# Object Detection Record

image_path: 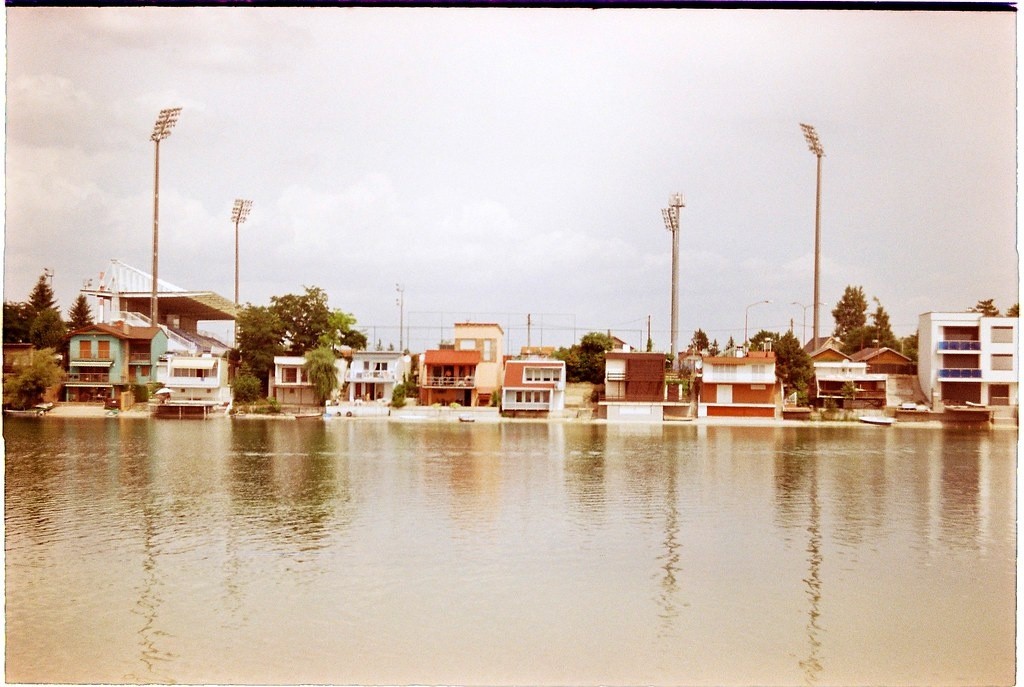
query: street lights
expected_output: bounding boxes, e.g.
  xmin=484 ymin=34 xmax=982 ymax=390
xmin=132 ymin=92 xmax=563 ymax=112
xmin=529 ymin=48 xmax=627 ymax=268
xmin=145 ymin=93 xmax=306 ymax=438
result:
xmin=662 ymin=191 xmax=687 ymax=373
xmin=792 ymin=302 xmax=831 ymax=347
xmin=146 ymin=105 xmax=183 ymax=326
xmin=798 ymin=119 xmax=828 ymax=355
xmin=744 ymin=300 xmax=773 ymax=356
xmin=394 ymin=281 xmax=406 ymax=353
xmin=231 ymin=197 xmax=255 ymax=309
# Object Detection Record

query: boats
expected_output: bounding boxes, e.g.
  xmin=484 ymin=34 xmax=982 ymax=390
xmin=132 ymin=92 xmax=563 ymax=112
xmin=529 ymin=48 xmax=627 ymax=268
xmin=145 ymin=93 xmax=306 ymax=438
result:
xmin=859 ymin=414 xmax=898 ymax=426
